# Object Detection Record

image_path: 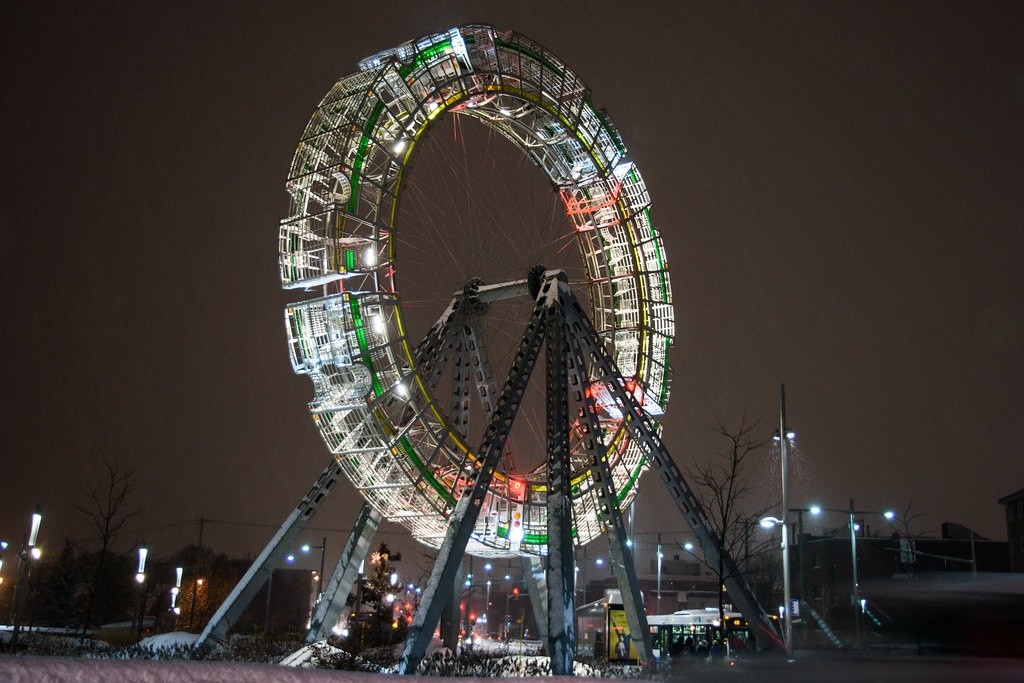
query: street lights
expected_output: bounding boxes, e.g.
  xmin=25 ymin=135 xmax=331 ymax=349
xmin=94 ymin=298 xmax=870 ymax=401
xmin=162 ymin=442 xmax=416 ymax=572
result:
xmin=656 ymin=533 xmax=665 ymax=616
xmin=755 ymin=380 xmax=796 ymax=663
xmin=9 ymin=532 xmax=43 ymax=655
xmin=128 ymin=557 xmax=152 ymax=645
xmin=188 ymin=570 xmax=204 ymax=634
xmin=808 ymin=499 xmax=898 ymax=643
xmin=302 ymin=537 xmax=328 ymax=602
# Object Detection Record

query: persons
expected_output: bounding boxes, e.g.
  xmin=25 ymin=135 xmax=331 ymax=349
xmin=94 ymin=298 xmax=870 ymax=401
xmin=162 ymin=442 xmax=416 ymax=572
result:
xmin=673 ymin=635 xmax=745 ymax=661
xmin=610 ymin=616 xmax=633 ymax=660
